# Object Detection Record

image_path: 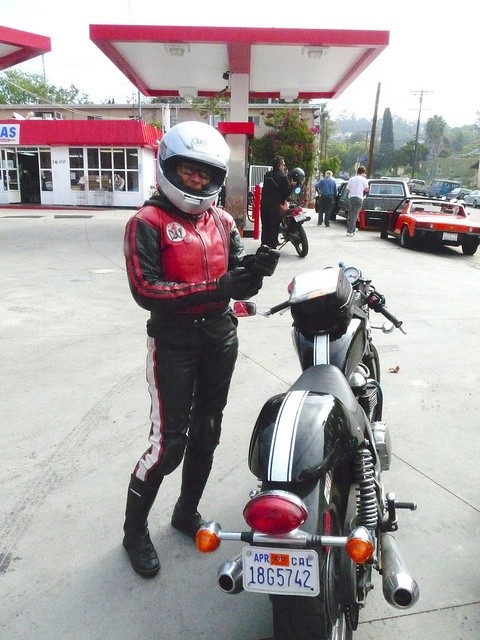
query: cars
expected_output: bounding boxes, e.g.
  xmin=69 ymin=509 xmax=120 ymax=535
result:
xmin=380 ymin=196 xmax=480 ymax=255
xmin=463 ymin=190 xmax=480 ymax=208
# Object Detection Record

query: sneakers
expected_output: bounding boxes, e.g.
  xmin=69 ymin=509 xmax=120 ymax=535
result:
xmin=353 ymin=228 xmax=358 ymax=235
xmin=317 ymin=223 xmax=322 ymax=225
xmin=326 ymin=226 xmax=330 ymax=227
xmin=346 ymin=233 xmax=353 ymax=237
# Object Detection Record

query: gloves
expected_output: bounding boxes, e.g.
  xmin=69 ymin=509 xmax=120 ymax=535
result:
xmin=216 ymin=267 xmax=264 ymax=301
xmin=243 ymin=245 xmax=281 ymax=276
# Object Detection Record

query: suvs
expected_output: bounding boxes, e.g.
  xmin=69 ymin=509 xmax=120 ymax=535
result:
xmin=330 ymin=179 xmax=410 ymax=228
xmin=408 ymin=179 xmax=426 ymax=196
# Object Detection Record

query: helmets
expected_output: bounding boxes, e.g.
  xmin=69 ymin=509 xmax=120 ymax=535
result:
xmin=289 ymin=167 xmax=305 ymax=187
xmin=156 ymin=121 xmax=231 ymax=214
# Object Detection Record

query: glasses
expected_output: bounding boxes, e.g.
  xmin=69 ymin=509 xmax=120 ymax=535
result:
xmin=179 ymin=161 xmax=214 ymax=180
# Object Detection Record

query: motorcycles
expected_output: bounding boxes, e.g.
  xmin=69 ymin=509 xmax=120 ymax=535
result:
xmin=278 ymin=186 xmax=311 ymax=258
xmin=196 ymin=262 xmax=420 ymax=636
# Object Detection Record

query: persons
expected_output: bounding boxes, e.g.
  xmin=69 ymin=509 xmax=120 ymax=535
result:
xmin=114 ymin=174 xmax=124 ymax=191
xmin=315 ymin=171 xmax=338 ymax=227
xmin=123 ymin=121 xmax=280 ymax=577
xmin=346 ymin=167 xmax=369 ymax=237
xmin=261 ymin=154 xmax=297 ymax=252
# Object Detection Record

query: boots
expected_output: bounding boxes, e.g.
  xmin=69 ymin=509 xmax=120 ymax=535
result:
xmin=123 ymin=474 xmax=164 ymax=576
xmin=171 ymin=447 xmax=214 ymax=538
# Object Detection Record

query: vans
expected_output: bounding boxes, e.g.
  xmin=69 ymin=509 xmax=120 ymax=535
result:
xmin=428 ymin=180 xmax=459 ymax=199
